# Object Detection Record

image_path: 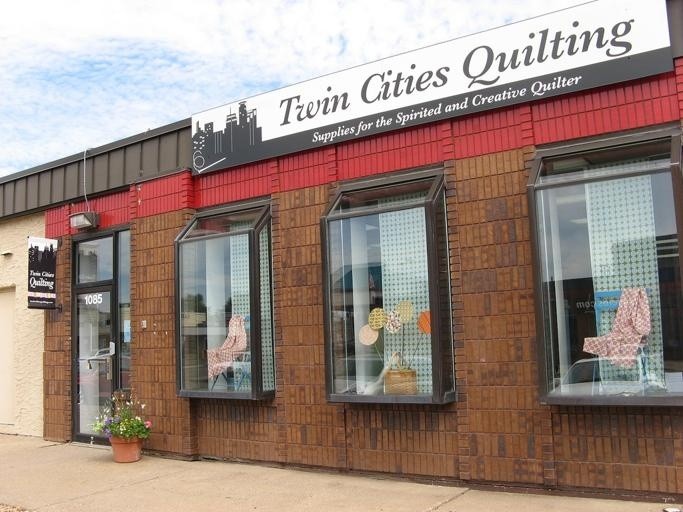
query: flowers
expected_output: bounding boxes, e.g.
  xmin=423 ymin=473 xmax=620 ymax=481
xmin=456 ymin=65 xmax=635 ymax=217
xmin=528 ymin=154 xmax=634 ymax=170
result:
xmin=92 ymin=395 xmax=152 ymax=441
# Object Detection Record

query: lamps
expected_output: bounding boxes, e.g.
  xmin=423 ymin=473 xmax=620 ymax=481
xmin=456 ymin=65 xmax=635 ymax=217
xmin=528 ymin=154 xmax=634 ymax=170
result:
xmin=68 ymin=211 xmax=96 ymax=232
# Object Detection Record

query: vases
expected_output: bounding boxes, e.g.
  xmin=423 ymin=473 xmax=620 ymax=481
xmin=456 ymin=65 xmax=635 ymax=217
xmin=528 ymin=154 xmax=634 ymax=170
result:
xmin=109 ymin=433 xmax=142 ymax=463
